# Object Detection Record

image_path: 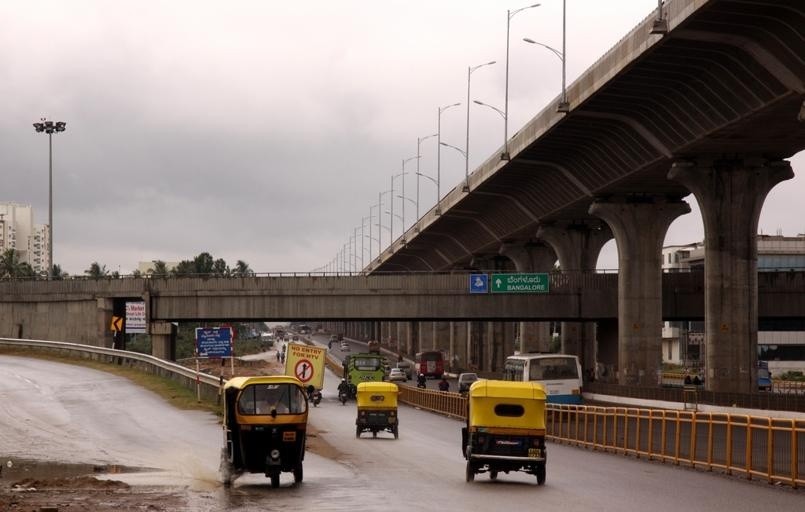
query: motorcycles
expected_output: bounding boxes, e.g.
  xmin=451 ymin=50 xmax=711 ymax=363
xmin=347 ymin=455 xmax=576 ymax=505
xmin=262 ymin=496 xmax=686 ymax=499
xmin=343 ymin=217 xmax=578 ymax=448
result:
xmin=337 ymin=387 xmax=347 ymax=405
xmin=312 ymin=390 xmax=322 ymax=407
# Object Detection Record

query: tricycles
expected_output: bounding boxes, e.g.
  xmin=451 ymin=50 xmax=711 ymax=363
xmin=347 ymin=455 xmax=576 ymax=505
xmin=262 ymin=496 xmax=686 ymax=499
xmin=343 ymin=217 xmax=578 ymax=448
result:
xmin=461 ymin=378 xmax=547 ymax=486
xmin=355 ymin=381 xmax=402 ymax=440
xmin=218 ymin=376 xmax=309 ymax=489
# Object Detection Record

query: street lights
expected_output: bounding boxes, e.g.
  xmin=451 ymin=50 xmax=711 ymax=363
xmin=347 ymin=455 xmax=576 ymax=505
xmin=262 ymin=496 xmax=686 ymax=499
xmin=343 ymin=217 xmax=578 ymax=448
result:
xmin=32 ymin=120 xmax=66 ymax=280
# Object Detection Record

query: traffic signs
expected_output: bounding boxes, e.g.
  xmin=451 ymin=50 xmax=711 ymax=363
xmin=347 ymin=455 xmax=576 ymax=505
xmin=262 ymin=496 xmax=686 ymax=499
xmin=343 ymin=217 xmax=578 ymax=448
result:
xmin=285 ymin=343 xmax=326 ymax=390
xmin=490 ymin=272 xmax=551 ymax=294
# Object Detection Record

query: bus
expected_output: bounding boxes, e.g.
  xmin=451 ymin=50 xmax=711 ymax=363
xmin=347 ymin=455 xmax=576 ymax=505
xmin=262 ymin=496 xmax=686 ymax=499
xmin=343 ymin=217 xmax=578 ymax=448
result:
xmin=501 ymin=353 xmax=583 ymax=418
xmin=342 ymin=352 xmax=385 ymax=400
xmin=415 ymin=349 xmax=444 ymax=380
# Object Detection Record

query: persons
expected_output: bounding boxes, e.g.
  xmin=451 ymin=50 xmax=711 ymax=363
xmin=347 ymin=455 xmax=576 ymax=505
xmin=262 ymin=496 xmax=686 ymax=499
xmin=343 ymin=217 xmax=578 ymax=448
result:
xmin=439 ymin=377 xmax=449 ymax=391
xmin=276 ymin=336 xmax=286 ymax=363
xmin=685 ymin=375 xmax=692 ymax=385
xmin=693 ymin=376 xmax=701 ymax=384
xmin=585 ymin=368 xmax=596 ymax=382
xmin=417 ymin=374 xmax=427 ymax=389
xmin=699 ymin=369 xmax=704 ymax=377
xmin=337 ymin=377 xmax=347 ymax=397
xmin=683 ymin=368 xmax=689 ymax=376
xmin=258 ymin=389 xmax=288 ymax=414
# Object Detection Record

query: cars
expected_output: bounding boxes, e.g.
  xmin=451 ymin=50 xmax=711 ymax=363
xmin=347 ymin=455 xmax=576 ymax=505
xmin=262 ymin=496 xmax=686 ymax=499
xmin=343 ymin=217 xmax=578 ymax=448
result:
xmin=383 ymin=356 xmax=413 ymax=383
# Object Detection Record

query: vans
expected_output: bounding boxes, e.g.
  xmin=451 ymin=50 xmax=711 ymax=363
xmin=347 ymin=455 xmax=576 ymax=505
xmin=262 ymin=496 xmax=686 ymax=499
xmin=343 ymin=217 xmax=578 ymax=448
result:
xmin=340 ymin=339 xmax=350 ymax=352
xmin=368 ymin=340 xmax=380 ymax=356
xmin=329 ymin=335 xmax=338 ymax=343
xmin=458 ymin=372 xmax=479 ymax=394
